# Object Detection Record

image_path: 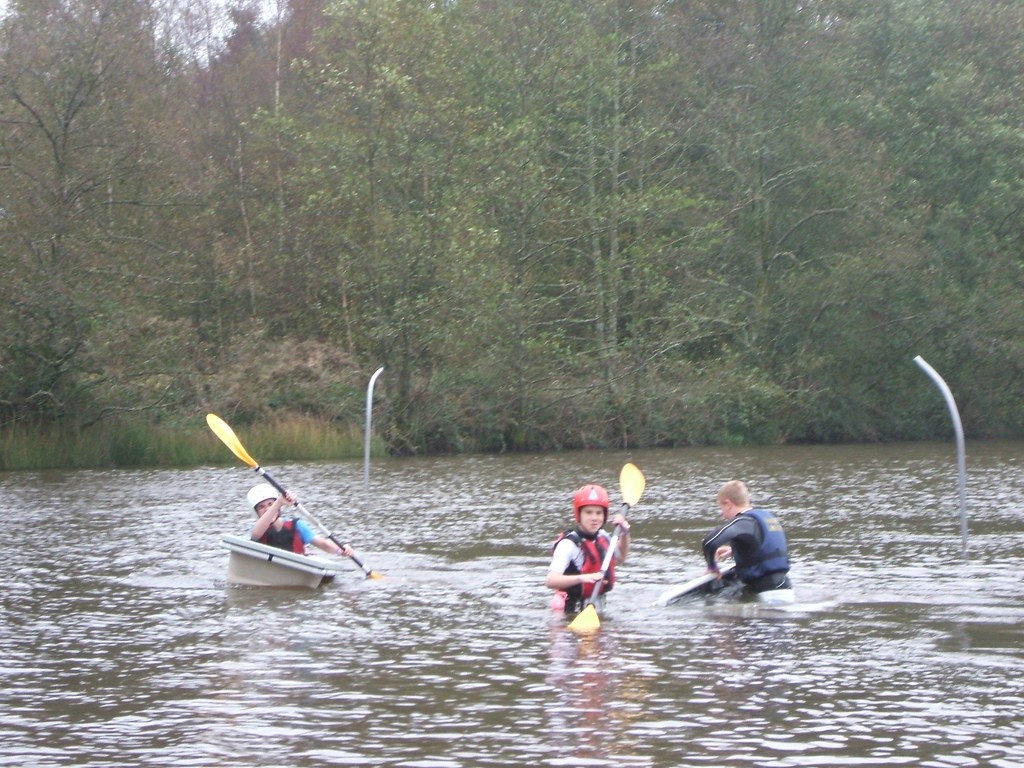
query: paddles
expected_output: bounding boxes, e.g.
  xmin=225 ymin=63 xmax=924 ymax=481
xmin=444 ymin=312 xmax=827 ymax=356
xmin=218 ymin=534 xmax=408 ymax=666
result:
xmin=567 ymin=463 xmax=646 ymax=635
xmin=205 ymin=411 xmax=381 ymax=579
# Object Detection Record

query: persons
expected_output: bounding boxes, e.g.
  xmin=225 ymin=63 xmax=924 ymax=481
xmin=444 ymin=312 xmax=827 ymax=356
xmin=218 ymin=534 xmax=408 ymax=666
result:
xmin=701 ymin=479 xmax=794 ymax=593
xmin=544 ymin=483 xmax=632 ymax=611
xmin=246 ymin=483 xmax=354 ymax=558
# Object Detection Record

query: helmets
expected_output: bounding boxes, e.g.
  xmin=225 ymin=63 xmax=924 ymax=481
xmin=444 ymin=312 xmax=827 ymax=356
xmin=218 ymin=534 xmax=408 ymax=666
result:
xmin=574 ymin=484 xmax=610 ymax=523
xmin=247 ymin=484 xmax=279 ymax=520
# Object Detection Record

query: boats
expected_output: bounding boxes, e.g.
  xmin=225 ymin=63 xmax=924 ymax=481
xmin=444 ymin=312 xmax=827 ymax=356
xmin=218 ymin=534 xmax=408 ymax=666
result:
xmin=647 ymin=559 xmax=837 ymax=618
xmin=218 ymin=533 xmax=344 ymax=592
